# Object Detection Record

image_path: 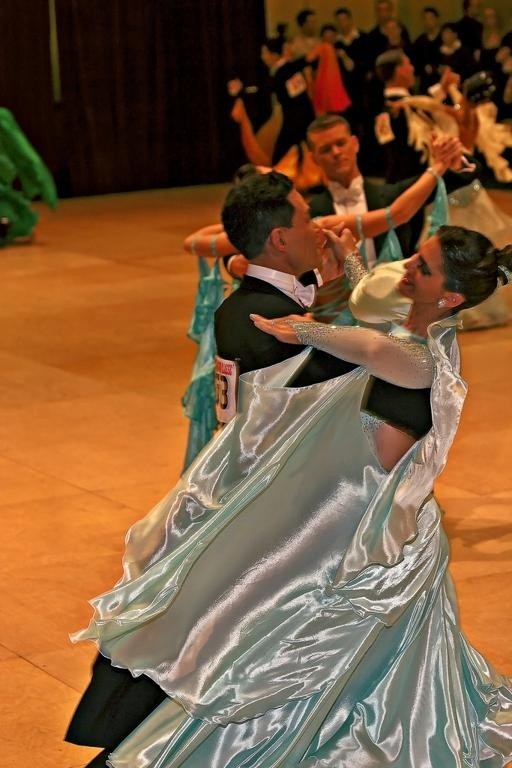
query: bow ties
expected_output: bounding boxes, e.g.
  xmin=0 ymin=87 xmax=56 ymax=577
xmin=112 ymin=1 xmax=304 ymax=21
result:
xmin=295 ymin=283 xmax=315 ymax=309
xmin=332 ymin=187 xmax=362 ymax=204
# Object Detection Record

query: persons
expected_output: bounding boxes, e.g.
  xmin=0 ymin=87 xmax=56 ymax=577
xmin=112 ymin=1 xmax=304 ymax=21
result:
xmin=181 ymin=1 xmax=511 ymax=473
xmin=63 ymin=172 xmax=346 ymax=766
xmin=69 ymin=223 xmax=511 ymax=766
